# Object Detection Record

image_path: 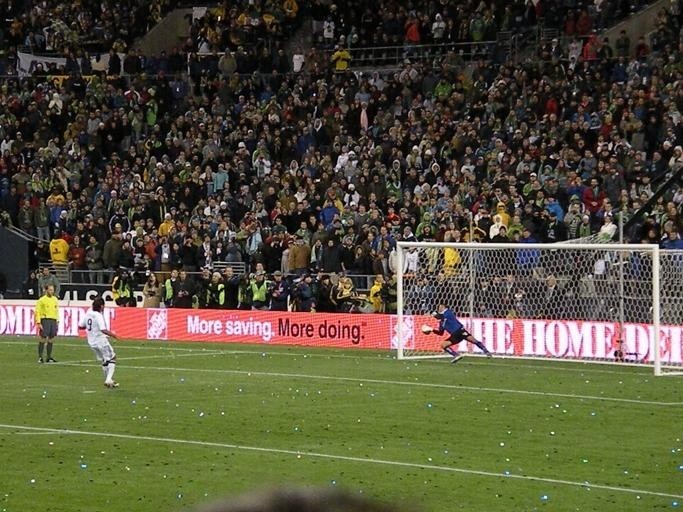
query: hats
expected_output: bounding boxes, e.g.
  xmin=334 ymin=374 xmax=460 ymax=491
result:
xmin=135 ymin=238 xmax=145 ymax=243
xmin=165 ymin=212 xmax=171 ymax=219
xmin=256 ymin=271 xmax=265 ymax=276
xmin=412 ymin=145 xmax=419 ymax=151
xmin=425 ymin=149 xmax=433 ymax=156
xmin=274 ymin=270 xmax=282 ymax=277
xmin=113 ymin=223 xmax=123 ymax=229
xmin=237 ymin=142 xmax=246 ymax=148
xmin=350 ymin=201 xmax=356 ymax=206
xmin=529 ymin=172 xmax=537 ymax=177
xmin=220 ymin=201 xmax=227 ymax=207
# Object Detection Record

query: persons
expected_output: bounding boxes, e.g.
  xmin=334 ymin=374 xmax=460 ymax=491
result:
xmin=0 ymin=2 xmax=682 ymax=324
xmin=77 ymin=298 xmax=124 ymax=388
xmin=34 ymin=283 xmax=60 ymax=364
xmin=428 ymin=303 xmax=492 ymax=364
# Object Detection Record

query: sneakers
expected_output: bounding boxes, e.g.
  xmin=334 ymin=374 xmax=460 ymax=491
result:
xmin=46 ymin=358 xmax=57 ymax=362
xmin=104 ymin=382 xmax=113 ymax=389
xmin=113 ymin=381 xmax=119 ymax=387
xmin=486 ymin=352 xmax=491 ymax=358
xmin=38 ymin=357 xmax=43 ymax=363
xmin=450 ymin=354 xmax=463 ymax=364
